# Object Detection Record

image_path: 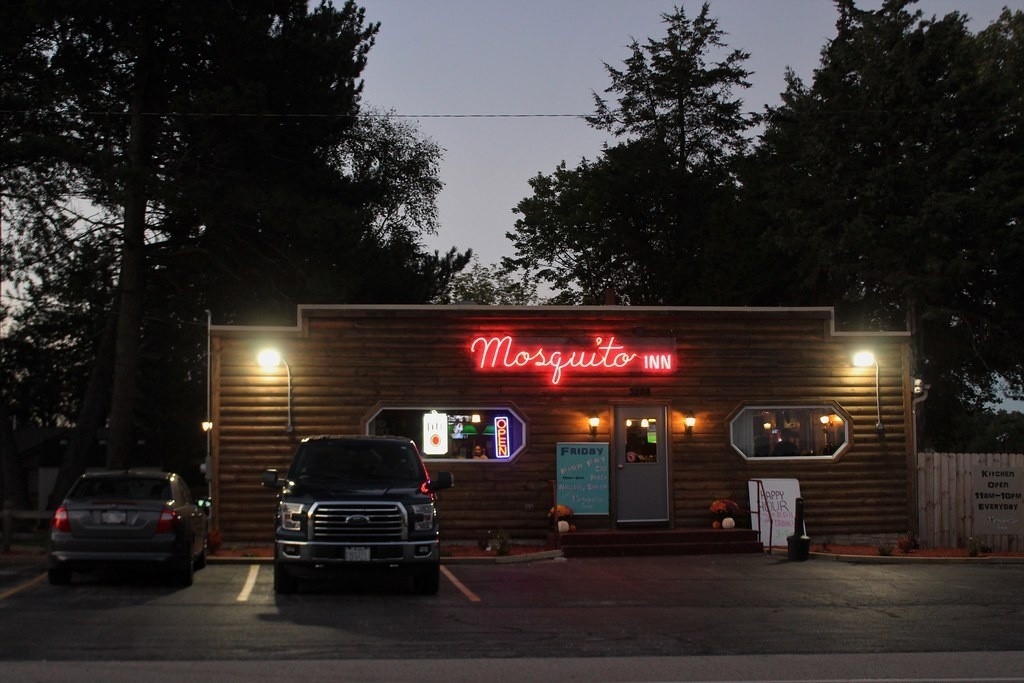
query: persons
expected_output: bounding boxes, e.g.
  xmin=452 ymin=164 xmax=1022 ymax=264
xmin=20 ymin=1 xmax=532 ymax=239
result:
xmin=769 ymin=428 xmax=799 ymax=456
xmin=472 ymin=445 xmax=488 ymax=460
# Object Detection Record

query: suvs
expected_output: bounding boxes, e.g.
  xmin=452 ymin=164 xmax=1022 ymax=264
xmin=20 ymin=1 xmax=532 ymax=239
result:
xmin=262 ymin=434 xmax=455 ymax=600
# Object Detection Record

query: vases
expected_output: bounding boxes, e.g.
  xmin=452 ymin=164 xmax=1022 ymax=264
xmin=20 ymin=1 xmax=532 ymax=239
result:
xmin=558 ymin=521 xmax=569 ymax=532
xmin=722 ymin=518 xmax=735 ymax=529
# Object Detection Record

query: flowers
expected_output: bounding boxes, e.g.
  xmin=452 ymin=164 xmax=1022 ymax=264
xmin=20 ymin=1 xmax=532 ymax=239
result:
xmin=549 ymin=506 xmax=573 ymax=519
xmin=710 ymin=499 xmax=738 ymax=515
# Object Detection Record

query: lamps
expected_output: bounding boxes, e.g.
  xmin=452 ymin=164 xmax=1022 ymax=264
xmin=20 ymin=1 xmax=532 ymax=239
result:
xmin=257 ymin=348 xmax=293 ymax=432
xmin=683 ymin=406 xmax=696 ymax=436
xmin=852 ymin=348 xmax=883 ymax=429
xmin=588 ymin=410 xmax=600 ymax=438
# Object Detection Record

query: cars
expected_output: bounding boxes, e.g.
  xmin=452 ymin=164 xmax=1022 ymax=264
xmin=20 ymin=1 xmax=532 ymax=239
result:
xmin=47 ymin=466 xmax=209 ymax=591
